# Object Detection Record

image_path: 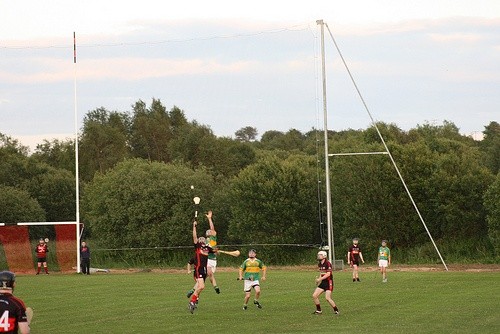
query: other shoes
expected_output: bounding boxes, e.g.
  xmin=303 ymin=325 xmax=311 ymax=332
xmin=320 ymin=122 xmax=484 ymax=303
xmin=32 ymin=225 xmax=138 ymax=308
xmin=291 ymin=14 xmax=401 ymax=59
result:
xmin=214 ymin=285 xmax=221 ymax=294
xmin=254 ymin=299 xmax=263 ymax=308
xmin=36 ymin=273 xmax=39 ymax=275
xmin=46 ymin=272 xmax=49 ymax=275
xmin=243 ymin=305 xmax=247 ymax=310
xmin=382 ymin=277 xmax=387 ymax=282
xmin=353 ymin=279 xmax=356 ymax=282
xmin=357 ymin=278 xmax=359 ymax=281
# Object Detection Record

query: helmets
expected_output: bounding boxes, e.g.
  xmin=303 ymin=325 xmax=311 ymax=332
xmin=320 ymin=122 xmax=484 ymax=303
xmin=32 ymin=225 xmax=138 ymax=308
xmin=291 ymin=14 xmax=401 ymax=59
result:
xmin=248 ymin=248 xmax=257 ymax=258
xmin=318 ymin=251 xmax=327 ymax=260
xmin=205 ymin=229 xmax=212 ymax=237
xmin=0 ymin=271 xmax=15 ymax=291
xmin=198 ymin=236 xmax=206 ymax=244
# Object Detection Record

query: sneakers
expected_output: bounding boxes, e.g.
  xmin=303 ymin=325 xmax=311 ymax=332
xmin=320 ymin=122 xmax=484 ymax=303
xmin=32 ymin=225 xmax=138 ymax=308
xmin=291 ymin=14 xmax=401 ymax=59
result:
xmin=186 ymin=288 xmax=195 ymax=298
xmin=188 ymin=301 xmax=198 ymax=314
xmin=313 ymin=310 xmax=323 ymax=314
xmin=334 ymin=310 xmax=338 ymax=314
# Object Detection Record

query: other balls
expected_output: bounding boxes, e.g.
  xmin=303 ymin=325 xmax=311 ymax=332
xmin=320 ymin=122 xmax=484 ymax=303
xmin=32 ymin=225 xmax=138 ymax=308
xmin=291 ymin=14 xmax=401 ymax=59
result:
xmin=191 ymin=185 xmax=194 ymax=189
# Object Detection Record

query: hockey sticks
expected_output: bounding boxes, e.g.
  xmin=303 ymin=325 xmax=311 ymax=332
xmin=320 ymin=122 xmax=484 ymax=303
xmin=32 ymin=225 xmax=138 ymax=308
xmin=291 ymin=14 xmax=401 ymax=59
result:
xmin=213 ymin=249 xmax=240 ymax=256
xmin=25 ymin=306 xmax=34 ymax=326
xmin=193 ymin=196 xmax=201 ymax=227
xmin=347 ymin=261 xmax=353 ymax=270
xmin=41 ymin=238 xmax=49 ymax=250
xmin=237 ymin=275 xmax=255 ymax=281
xmin=315 ymin=276 xmax=321 ymax=286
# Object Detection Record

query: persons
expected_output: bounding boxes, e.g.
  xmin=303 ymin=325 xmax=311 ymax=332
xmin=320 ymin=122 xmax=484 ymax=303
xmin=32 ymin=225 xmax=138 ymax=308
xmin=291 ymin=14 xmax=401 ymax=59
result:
xmin=187 ymin=211 xmax=220 ymax=298
xmin=80 ymin=241 xmax=90 ymax=275
xmin=188 ymin=221 xmax=220 ymax=314
xmin=239 ymin=249 xmax=266 ymax=311
xmin=0 ymin=271 xmax=31 ymax=334
xmin=348 ymin=238 xmax=364 ymax=283
xmin=377 ymin=240 xmax=390 ymax=282
xmin=35 ymin=237 xmax=50 ymax=275
xmin=311 ymin=251 xmax=340 ymax=316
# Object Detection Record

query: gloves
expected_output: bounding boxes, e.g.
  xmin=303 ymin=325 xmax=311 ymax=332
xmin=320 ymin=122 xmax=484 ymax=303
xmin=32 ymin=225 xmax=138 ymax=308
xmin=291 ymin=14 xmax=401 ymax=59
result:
xmin=25 ymin=307 xmax=34 ymax=325
